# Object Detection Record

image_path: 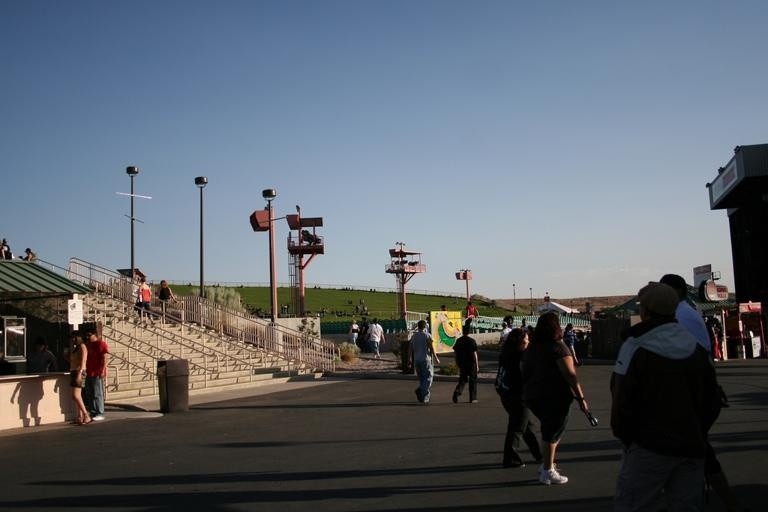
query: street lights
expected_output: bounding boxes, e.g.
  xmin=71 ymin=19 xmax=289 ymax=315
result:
xmin=261 ymin=188 xmax=277 ymax=324
xmin=193 ymin=175 xmax=209 ymax=298
xmin=529 ymin=287 xmax=534 ymax=316
xmin=126 ymin=166 xmax=139 ymax=278
xmin=512 ymin=283 xmax=517 ymax=313
xmin=392 ymin=260 xmax=419 ymax=322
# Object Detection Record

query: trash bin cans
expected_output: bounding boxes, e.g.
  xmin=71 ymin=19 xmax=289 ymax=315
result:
xmin=400 ymin=340 xmax=415 ymax=374
xmin=156 ymin=359 xmax=189 ymax=412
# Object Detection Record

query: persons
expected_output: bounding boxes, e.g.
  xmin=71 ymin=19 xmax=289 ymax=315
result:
xmin=25 ymin=245 xmax=38 ymax=264
xmin=344 ymin=294 xmax=612 ymax=369
xmin=60 ymin=332 xmax=92 ymax=427
xmin=452 ymin=324 xmax=484 ymax=404
xmin=157 ymin=279 xmax=179 ymax=321
xmin=25 ymin=335 xmax=59 ymax=372
xmin=2 ymin=237 xmax=13 ymax=262
xmin=405 ymin=319 xmax=442 ymax=407
xmin=133 ymin=277 xmax=156 ymax=329
xmin=608 ymin=281 xmax=724 ymax=511
xmin=659 ymin=271 xmax=740 ymax=511
xmin=84 ymin=327 xmax=110 ymax=422
xmin=492 ymin=327 xmax=543 ymax=469
xmin=521 ymin=309 xmax=599 ymax=487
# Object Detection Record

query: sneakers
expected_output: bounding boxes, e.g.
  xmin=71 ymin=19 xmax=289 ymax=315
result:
xmin=537 ymin=462 xmax=569 ymax=485
xmin=93 ymin=416 xmax=106 ymax=421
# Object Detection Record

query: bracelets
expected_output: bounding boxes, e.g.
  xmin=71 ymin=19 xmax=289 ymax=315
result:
xmin=580 ymin=397 xmax=585 ymax=402
